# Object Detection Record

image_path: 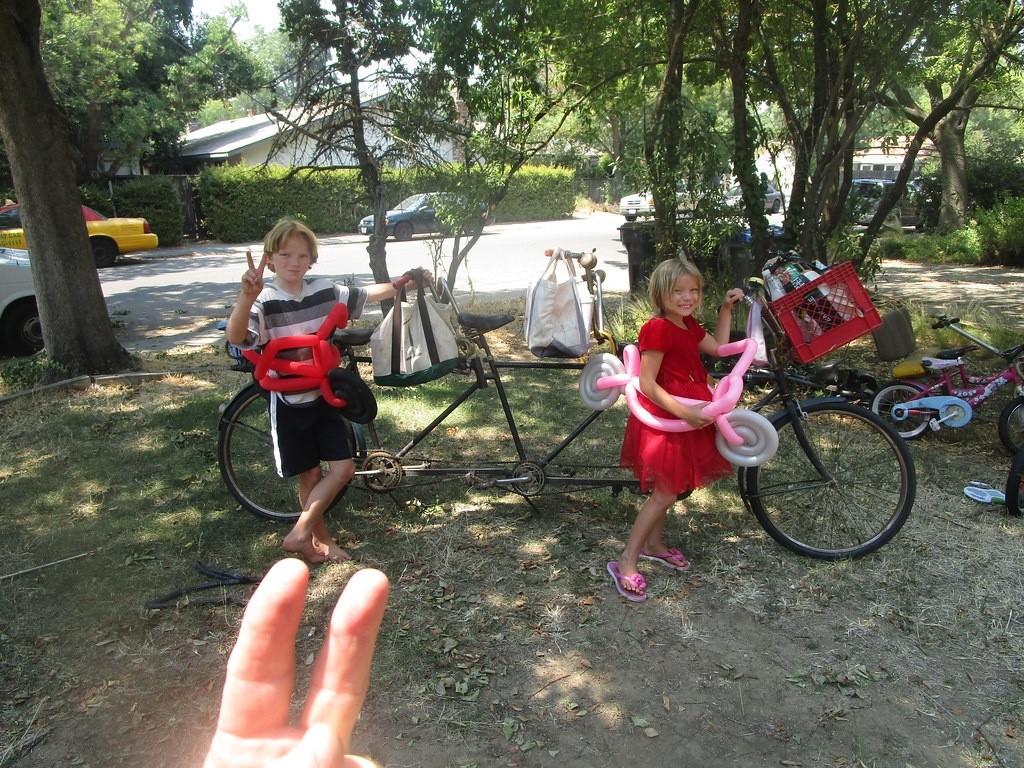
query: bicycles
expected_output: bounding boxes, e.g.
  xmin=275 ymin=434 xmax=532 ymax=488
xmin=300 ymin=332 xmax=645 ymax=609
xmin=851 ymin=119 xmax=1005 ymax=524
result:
xmin=213 ymin=268 xmax=919 ymax=560
xmin=869 ymin=343 xmax=1024 ymax=519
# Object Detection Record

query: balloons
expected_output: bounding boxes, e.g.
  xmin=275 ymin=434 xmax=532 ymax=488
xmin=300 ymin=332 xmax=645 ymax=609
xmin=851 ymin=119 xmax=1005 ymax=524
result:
xmin=579 ymin=338 xmax=779 ymax=468
xmin=241 ymin=302 xmax=377 ymax=425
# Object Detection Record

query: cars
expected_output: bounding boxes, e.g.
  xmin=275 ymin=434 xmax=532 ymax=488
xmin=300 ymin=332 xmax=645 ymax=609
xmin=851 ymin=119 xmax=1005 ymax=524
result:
xmin=619 ymin=176 xmax=722 ymax=221
xmin=0 ymin=197 xmax=160 ymax=269
xmin=726 ymin=183 xmax=784 ymax=217
xmin=359 ymin=189 xmax=496 ymax=243
xmin=0 ymin=247 xmax=47 ymax=359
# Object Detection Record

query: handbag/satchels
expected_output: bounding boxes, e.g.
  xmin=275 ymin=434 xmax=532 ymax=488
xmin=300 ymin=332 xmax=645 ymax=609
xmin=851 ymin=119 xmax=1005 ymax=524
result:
xmin=522 ymin=247 xmax=594 ymax=357
xmin=371 ymin=269 xmax=460 ymax=386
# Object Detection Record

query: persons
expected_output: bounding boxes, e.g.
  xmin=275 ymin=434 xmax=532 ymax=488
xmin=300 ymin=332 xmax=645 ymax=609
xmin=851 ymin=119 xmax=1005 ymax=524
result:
xmin=226 ymin=220 xmax=436 ymax=563
xmin=201 ymin=557 xmax=390 ymax=768
xmin=618 ymin=260 xmax=746 ymax=595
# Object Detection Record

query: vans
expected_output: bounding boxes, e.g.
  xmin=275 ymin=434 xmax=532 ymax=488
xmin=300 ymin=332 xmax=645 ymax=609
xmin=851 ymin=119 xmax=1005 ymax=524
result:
xmin=843 ymin=177 xmax=930 ymax=234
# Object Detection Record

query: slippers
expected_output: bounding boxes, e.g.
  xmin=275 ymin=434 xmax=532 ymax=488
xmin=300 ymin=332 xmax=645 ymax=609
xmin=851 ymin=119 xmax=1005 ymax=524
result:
xmin=638 ymin=547 xmax=690 ymax=571
xmin=606 ymin=561 xmax=647 ymax=602
